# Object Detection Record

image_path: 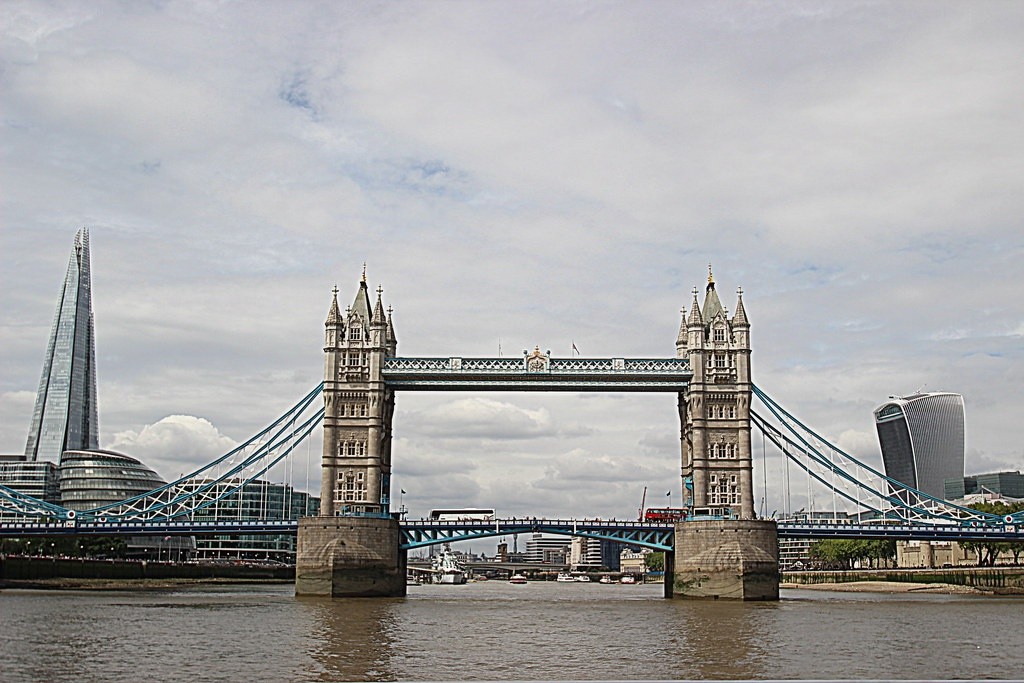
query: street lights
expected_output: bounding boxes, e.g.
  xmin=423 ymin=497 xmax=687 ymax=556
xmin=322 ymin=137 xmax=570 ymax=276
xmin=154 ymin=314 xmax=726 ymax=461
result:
xmin=27 ymin=541 xmax=32 ymax=558
xmin=51 ymin=542 xmax=55 ymax=559
xmin=143 ymin=549 xmax=290 ymax=564
xmin=111 ymin=547 xmax=115 ymax=562
xmin=80 ymin=545 xmax=84 ymax=560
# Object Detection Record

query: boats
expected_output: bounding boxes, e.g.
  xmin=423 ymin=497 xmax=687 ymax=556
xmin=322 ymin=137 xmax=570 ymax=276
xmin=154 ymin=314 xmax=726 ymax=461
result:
xmin=432 ymin=545 xmax=468 ymax=584
xmin=556 ymin=572 xmax=636 ymax=584
xmin=509 ymin=573 xmax=527 ymax=584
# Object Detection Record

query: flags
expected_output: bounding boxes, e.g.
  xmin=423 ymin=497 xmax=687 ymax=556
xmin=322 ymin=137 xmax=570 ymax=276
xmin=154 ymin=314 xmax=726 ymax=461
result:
xmin=667 ymin=492 xmax=670 ymax=496
xmin=573 ymin=344 xmax=579 ymax=354
xmin=401 ymin=489 xmax=406 ymax=494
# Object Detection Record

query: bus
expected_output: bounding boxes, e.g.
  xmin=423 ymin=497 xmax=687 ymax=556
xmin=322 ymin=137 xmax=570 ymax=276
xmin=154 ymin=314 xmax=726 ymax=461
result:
xmin=645 ymin=507 xmax=690 ymax=523
xmin=428 ymin=508 xmax=496 ymax=525
